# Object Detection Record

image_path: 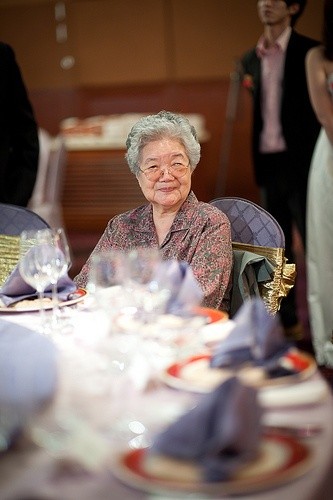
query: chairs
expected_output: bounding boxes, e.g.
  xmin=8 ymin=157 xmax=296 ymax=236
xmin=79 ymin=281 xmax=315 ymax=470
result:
xmin=207 ymin=196 xmax=286 ymax=321
xmin=0 ymin=204 xmax=52 ymax=289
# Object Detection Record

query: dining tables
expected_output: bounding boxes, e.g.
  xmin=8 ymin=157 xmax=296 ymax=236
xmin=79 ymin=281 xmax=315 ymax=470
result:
xmin=0 ymin=292 xmax=333 ymax=500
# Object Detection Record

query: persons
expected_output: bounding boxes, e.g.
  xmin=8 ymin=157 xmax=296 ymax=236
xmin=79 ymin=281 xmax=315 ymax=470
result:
xmin=72 ymin=110 xmax=232 ymax=311
xmin=0 ymin=42 xmax=40 ymax=208
xmin=240 ymin=0 xmax=321 ymax=327
xmin=306 ymin=0 xmax=333 ymax=365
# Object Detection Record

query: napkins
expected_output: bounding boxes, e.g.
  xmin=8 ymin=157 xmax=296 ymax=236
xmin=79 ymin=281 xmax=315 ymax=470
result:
xmin=149 ymin=258 xmax=206 ymax=316
xmin=207 ymin=296 xmax=296 ymax=367
xmin=148 ymin=373 xmax=272 ymax=483
xmin=0 ymin=241 xmax=77 ymax=306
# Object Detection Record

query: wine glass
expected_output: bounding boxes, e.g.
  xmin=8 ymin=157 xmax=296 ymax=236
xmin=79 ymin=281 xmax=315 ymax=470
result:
xmin=83 ymin=246 xmax=186 ymax=424
xmin=18 ymin=226 xmax=74 ymax=326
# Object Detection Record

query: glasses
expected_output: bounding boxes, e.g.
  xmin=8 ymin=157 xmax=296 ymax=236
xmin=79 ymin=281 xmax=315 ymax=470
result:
xmin=135 ymin=156 xmax=189 ymax=182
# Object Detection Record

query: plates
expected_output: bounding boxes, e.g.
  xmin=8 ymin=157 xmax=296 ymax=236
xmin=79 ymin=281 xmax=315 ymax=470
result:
xmin=196 ymin=306 xmax=229 ymax=326
xmin=161 ymin=346 xmax=318 ymax=396
xmin=106 ymin=431 xmax=317 ymax=496
xmin=1 ymin=285 xmax=85 ymax=313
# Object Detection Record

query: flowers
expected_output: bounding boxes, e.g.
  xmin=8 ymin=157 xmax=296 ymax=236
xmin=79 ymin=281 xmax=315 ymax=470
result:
xmin=243 ymin=74 xmax=253 ymax=89
